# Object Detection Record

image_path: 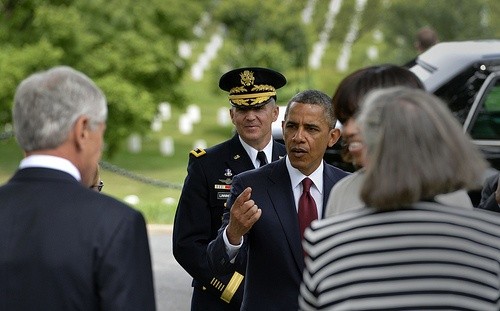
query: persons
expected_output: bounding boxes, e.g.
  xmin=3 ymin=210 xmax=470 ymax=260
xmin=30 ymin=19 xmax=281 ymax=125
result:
xmin=299 ymin=63 xmax=500 ymax=311
xmin=0 ymin=66 xmax=156 ymax=311
xmin=205 ymin=89 xmax=354 ymax=311
xmin=87 ymin=161 xmax=103 ymax=193
xmin=403 ymin=26 xmax=441 ymax=69
xmin=170 ymin=66 xmax=288 ymax=311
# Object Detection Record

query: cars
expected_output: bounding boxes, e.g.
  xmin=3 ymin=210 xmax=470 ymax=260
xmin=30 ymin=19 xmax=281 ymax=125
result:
xmin=272 ymin=38 xmax=500 ymax=209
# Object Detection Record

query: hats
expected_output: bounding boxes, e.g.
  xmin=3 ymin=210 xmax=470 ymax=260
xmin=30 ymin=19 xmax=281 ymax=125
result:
xmin=218 ymin=66 xmax=287 ymax=110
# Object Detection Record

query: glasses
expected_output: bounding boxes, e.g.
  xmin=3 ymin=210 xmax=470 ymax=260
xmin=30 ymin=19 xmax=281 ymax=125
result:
xmin=89 ymin=179 xmax=104 ymax=192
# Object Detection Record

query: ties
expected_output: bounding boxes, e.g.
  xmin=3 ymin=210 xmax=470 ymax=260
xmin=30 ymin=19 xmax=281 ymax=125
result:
xmin=256 ymin=151 xmax=268 ymax=169
xmin=297 ymin=178 xmax=319 ymax=258
